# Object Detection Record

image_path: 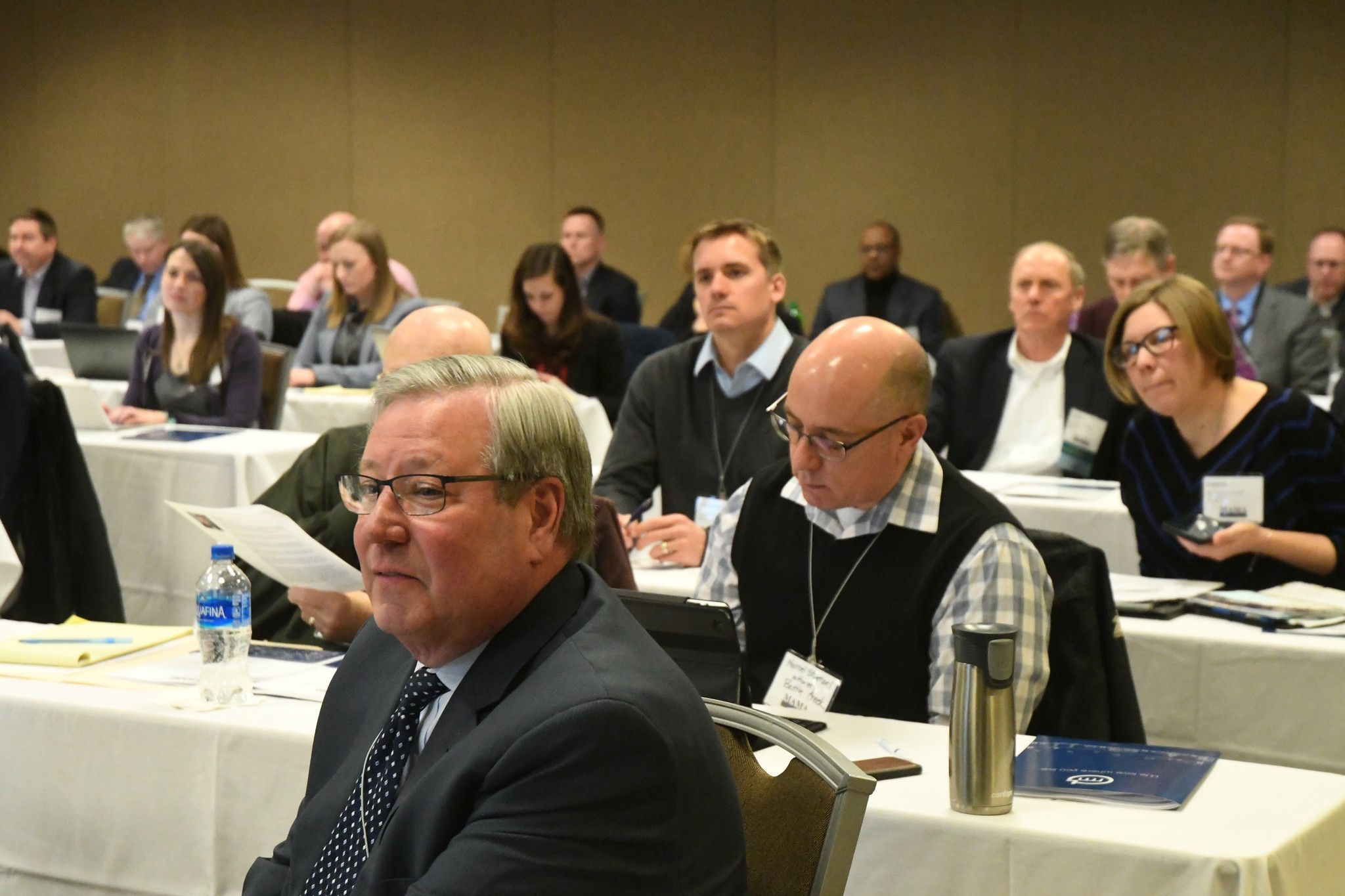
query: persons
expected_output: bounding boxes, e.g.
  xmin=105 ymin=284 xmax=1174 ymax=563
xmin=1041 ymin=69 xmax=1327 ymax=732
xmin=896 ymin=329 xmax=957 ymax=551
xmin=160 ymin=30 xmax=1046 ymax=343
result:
xmin=654 ymin=274 xmax=804 ymax=342
xmin=116 ymin=208 xmax=427 ymax=388
xmin=594 ymin=219 xmax=812 ymax=568
xmin=501 ymin=239 xmax=618 ymax=413
xmin=1078 ymin=214 xmax=1258 ymax=384
xmin=557 ymin=206 xmax=641 ymax=322
xmin=241 ymin=354 xmax=747 ymax=896
xmin=1102 ymin=273 xmax=1345 ymax=593
xmin=924 ymin=239 xmax=1127 ymax=484
xmin=690 ymin=315 xmax=1053 ymax=739
xmin=808 ymin=220 xmax=961 ymax=353
xmin=101 ymin=238 xmax=263 ymax=426
xmin=0 ymin=342 xmax=77 ymax=623
xmin=0 ymin=209 xmax=98 ymax=339
xmin=1206 ymin=216 xmax=1345 ymax=397
xmin=233 ymin=306 xmax=494 ymax=654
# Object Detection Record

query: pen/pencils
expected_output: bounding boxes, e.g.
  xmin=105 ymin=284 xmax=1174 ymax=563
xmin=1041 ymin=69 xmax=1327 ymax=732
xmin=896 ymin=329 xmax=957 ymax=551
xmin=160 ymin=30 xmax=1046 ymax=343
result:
xmin=1211 ymin=604 xmax=1273 ymax=626
xmin=622 ymin=498 xmax=654 ymax=529
xmin=18 ymin=636 xmax=131 ymax=643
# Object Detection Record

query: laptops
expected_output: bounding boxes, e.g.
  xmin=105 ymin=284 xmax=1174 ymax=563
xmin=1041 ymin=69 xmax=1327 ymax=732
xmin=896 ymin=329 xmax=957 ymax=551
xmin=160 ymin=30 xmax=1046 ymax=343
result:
xmin=614 ymin=587 xmax=826 ymax=752
xmin=61 ymin=323 xmax=140 ymax=380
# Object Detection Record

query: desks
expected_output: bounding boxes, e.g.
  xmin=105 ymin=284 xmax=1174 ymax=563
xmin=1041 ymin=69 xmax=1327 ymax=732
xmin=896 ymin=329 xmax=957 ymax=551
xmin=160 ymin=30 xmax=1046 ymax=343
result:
xmin=0 ymin=337 xmax=1345 ymax=896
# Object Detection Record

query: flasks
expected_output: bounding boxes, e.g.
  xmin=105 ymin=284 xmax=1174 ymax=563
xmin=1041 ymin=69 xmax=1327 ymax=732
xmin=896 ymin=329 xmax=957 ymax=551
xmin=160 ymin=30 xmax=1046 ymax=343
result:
xmin=948 ymin=621 xmax=1021 ymax=816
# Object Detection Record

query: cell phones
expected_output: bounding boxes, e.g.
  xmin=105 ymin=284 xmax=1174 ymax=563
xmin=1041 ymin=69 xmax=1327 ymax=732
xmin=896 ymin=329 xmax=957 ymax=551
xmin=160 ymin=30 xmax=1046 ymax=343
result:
xmin=1160 ymin=521 xmax=1227 ymax=542
xmin=854 ymin=757 xmax=922 ymax=781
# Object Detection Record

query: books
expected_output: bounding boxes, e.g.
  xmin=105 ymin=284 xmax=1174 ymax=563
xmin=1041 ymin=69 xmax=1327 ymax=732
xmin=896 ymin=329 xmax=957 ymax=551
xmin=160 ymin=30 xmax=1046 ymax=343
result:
xmin=1010 ymin=733 xmax=1222 ymax=810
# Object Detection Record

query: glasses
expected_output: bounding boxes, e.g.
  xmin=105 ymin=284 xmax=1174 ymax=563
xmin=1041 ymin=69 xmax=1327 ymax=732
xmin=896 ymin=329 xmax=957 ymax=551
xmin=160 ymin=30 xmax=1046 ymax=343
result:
xmin=1109 ymin=326 xmax=1178 ymax=369
xmin=766 ymin=391 xmax=910 ymax=463
xmin=335 ymin=473 xmax=528 ymax=517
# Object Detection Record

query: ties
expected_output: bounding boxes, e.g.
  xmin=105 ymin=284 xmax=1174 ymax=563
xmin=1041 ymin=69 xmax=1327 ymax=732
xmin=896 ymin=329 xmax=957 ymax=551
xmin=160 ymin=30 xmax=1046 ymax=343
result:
xmin=300 ymin=667 xmax=450 ymax=895
xmin=1224 ymin=308 xmax=1243 ymax=330
xmin=129 ymin=272 xmax=155 ymax=320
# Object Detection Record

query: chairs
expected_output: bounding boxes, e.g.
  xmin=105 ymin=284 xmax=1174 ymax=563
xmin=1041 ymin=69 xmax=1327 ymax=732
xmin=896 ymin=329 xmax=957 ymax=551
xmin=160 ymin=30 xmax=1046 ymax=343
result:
xmin=257 ymin=341 xmax=297 ymax=430
xmin=1024 ymin=528 xmax=1147 ymax=745
xmin=700 ymin=697 xmax=877 ymax=896
xmin=273 ymin=308 xmax=311 ymax=348
xmin=95 ymin=284 xmax=129 ymax=329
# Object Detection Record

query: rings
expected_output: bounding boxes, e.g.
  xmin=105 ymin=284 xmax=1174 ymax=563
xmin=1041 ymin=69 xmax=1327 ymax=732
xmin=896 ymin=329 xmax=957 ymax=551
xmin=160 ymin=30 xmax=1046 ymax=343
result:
xmin=307 ymin=617 xmax=315 ymax=626
xmin=661 ymin=542 xmax=669 ymax=554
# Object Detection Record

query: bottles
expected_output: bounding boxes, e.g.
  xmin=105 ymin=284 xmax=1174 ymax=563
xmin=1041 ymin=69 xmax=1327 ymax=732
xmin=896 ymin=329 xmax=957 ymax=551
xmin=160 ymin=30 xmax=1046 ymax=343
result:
xmin=787 ymin=301 xmax=803 ymax=327
xmin=194 ymin=544 xmax=255 ymax=707
